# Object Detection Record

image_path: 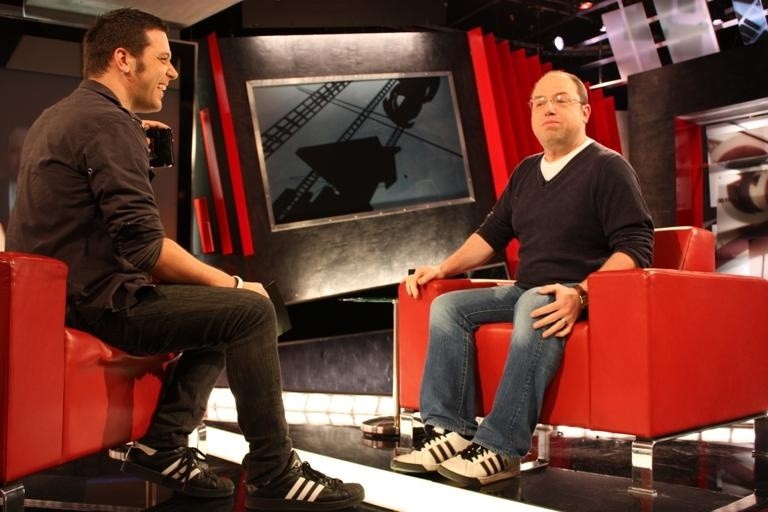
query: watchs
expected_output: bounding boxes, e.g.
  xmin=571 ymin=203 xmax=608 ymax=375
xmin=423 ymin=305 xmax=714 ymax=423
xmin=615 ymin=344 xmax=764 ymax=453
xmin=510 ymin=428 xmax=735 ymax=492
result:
xmin=570 ymin=284 xmax=588 ymax=310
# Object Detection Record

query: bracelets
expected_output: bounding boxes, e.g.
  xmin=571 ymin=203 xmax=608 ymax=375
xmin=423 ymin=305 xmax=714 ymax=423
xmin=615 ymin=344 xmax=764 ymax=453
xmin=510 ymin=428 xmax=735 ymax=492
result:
xmin=232 ymin=276 xmax=244 ymax=290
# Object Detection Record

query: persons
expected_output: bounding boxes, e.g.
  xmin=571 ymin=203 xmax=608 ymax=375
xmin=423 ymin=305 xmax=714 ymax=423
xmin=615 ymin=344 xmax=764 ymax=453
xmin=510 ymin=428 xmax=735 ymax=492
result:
xmin=390 ymin=70 xmax=655 ymax=487
xmin=4 ymin=8 xmax=364 ymax=511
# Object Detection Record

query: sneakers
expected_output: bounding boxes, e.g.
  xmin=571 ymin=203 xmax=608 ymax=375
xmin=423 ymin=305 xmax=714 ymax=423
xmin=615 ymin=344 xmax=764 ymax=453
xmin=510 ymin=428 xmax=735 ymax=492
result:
xmin=390 ymin=425 xmax=473 ymax=473
xmin=244 ymin=450 xmax=364 ymax=510
xmin=437 ymin=442 xmax=521 ymax=486
xmin=120 ymin=440 xmax=236 ymax=498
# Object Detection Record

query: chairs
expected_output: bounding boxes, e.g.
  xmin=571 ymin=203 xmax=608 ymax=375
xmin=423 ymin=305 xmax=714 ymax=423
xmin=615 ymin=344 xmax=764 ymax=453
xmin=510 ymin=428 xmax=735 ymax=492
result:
xmin=395 ymin=221 xmax=767 ymax=498
xmin=0 ymin=242 xmax=183 ymax=512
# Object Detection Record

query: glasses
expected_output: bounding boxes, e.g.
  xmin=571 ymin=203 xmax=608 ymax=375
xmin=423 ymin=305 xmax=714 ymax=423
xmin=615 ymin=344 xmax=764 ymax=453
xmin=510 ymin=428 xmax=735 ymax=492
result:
xmin=528 ymin=94 xmax=585 ymax=110
